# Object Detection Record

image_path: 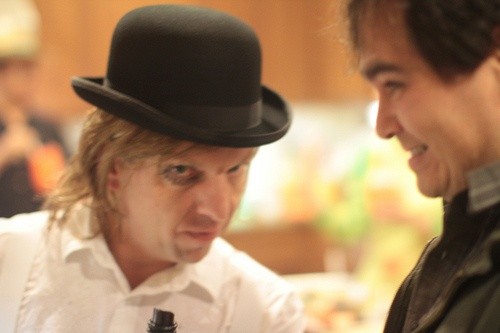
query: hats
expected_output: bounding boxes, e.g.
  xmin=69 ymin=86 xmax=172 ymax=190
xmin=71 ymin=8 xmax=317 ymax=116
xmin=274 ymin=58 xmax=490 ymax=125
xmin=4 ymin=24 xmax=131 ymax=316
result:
xmin=71 ymin=3 xmax=294 ymax=147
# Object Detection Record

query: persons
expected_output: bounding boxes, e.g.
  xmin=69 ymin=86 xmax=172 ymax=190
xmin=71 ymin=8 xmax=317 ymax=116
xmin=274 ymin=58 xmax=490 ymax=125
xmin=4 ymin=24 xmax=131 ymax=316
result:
xmin=0 ymin=1 xmax=75 ymax=218
xmin=2 ymin=6 xmax=308 ymax=333
xmin=345 ymin=0 xmax=500 ymax=333
